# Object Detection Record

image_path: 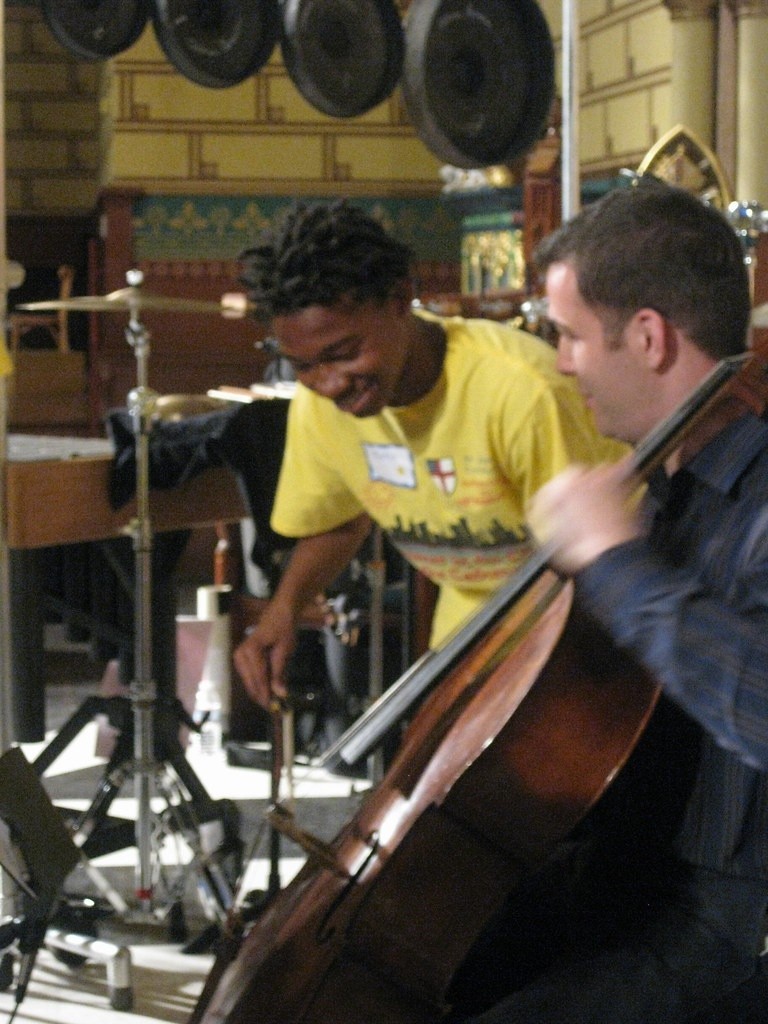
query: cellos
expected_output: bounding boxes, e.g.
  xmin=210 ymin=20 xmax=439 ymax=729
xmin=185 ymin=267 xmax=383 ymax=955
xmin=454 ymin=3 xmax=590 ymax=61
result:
xmin=191 ymin=313 xmax=767 ymax=1021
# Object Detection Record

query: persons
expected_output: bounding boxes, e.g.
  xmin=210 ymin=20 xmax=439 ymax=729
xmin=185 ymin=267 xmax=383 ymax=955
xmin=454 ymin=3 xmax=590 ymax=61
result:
xmin=432 ymin=178 xmax=768 ymax=1024
xmin=228 ymin=199 xmax=654 ymax=720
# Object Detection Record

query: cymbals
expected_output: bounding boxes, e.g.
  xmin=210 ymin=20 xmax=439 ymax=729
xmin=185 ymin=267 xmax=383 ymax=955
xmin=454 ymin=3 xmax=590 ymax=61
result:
xmin=16 ymin=287 xmax=236 ymax=312
